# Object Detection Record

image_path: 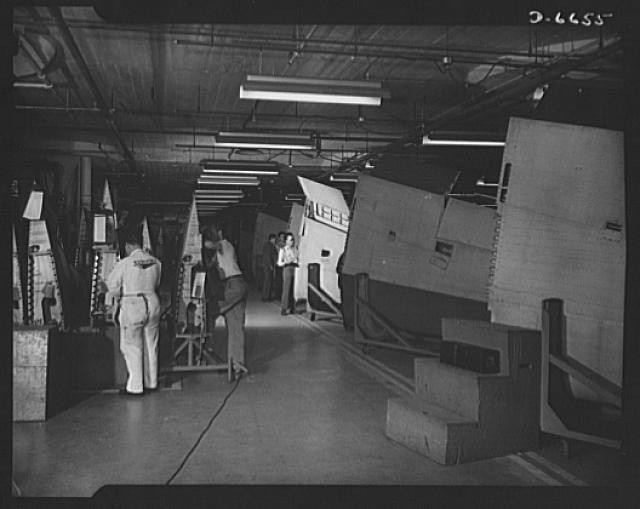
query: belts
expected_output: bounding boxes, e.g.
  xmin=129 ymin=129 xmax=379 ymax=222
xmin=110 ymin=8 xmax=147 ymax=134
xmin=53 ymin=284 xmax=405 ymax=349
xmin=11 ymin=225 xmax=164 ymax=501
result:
xmin=223 ymin=275 xmax=245 ymax=280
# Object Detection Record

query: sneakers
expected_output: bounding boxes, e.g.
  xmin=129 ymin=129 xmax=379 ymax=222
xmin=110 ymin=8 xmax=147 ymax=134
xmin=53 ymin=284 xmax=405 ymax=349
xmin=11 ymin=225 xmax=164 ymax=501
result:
xmin=119 ymin=386 xmax=157 ymax=395
xmin=281 ymin=309 xmax=287 ymax=315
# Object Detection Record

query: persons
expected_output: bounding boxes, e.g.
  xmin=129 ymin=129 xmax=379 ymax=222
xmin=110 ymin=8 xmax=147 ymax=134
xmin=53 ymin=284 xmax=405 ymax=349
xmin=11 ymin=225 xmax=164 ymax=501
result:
xmin=261 ymin=234 xmax=278 ymax=302
xmin=104 ymin=232 xmax=162 ymax=397
xmin=277 ymin=233 xmax=298 ymax=315
xmin=202 ymin=220 xmax=248 ymax=380
xmin=273 ymin=231 xmax=287 ymax=300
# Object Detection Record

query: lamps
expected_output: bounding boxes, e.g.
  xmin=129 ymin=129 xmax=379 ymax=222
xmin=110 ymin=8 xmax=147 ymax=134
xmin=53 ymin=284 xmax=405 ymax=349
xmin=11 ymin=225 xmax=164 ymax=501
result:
xmin=213 ymin=132 xmax=317 ymax=152
xmin=239 ymin=75 xmax=392 ymax=107
xmin=194 ymin=159 xmax=280 ymax=217
xmin=422 ymin=129 xmax=506 ymax=148
